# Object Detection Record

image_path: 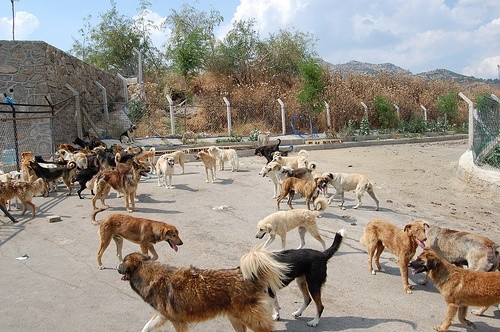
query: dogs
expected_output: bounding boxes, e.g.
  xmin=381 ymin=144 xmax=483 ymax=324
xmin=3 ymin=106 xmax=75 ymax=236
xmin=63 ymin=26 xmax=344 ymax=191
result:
xmin=90 ymin=209 xmax=183 ymax=270
xmin=407 ymin=246 xmax=500 ymax=332
xmin=0 ymin=174 xmax=44 ymax=217
xmin=258 ymin=128 xmax=271 ymax=147
xmin=276 ymin=177 xmax=328 ymax=211
xmin=120 ymin=125 xmax=137 ymax=145
xmin=259 ymin=164 xmax=278 ymax=199
xmin=208 ymin=146 xmax=239 ymax=172
xmin=86 ymin=161 xmax=150 ymax=213
xmin=268 ymin=229 xmax=345 ymax=327
xmin=321 ymin=172 xmax=379 ymax=211
xmin=265 ymin=162 xmax=316 ymax=199
xmin=54 ymin=136 xmax=155 ymax=199
xmin=115 ymin=239 xmax=294 ymax=332
xmin=159 ymin=149 xmax=189 ymax=175
xmin=34 ymin=155 xmax=68 ymax=168
xmin=182 ymin=130 xmax=197 ymax=147
xmin=255 ymin=196 xmax=329 ymax=252
xmin=38 ymin=163 xmax=57 ymax=193
xmin=194 ymin=150 xmax=217 ymax=184
xmin=360 ymin=218 xmax=430 ymax=294
xmin=417 ymin=224 xmax=500 ymax=285
xmin=27 ymin=161 xmax=77 ymax=196
xmin=154 ymin=157 xmax=175 ymax=189
xmin=20 ymin=152 xmax=38 ymax=184
xmin=272 ymin=150 xmax=310 ymax=170
xmin=255 ymin=138 xmax=281 ymax=162
xmin=268 ymin=145 xmax=293 ymax=161
xmin=271 ymin=151 xmax=286 ymax=160
